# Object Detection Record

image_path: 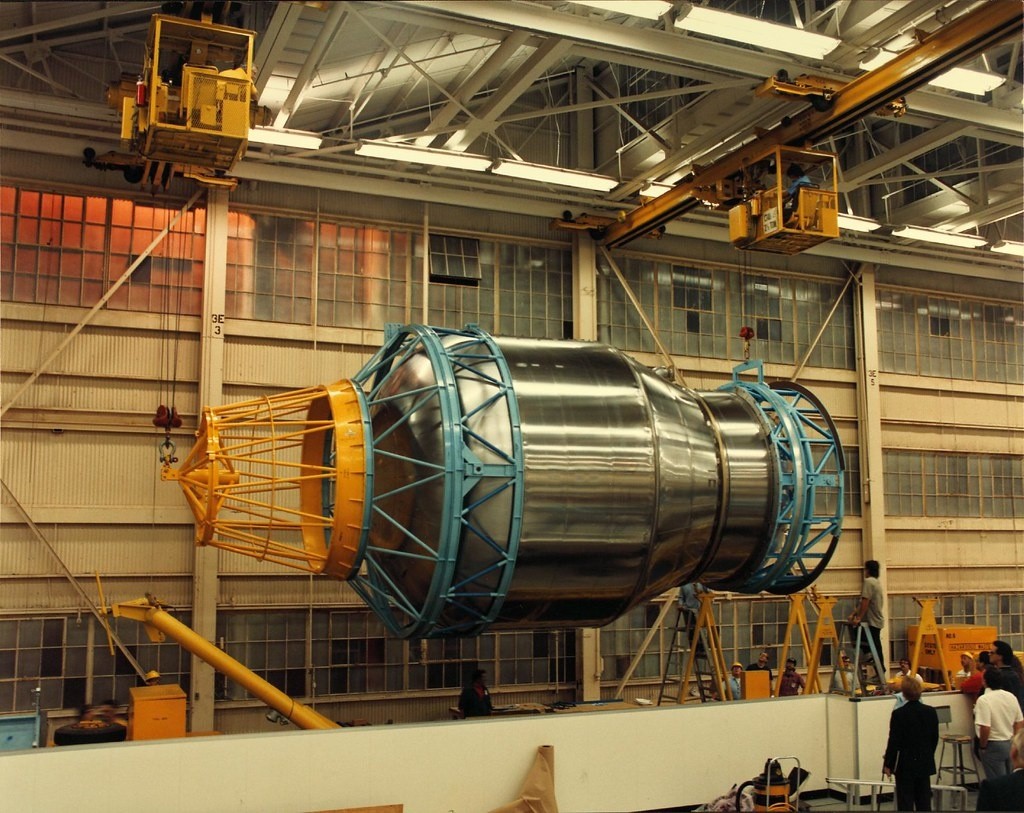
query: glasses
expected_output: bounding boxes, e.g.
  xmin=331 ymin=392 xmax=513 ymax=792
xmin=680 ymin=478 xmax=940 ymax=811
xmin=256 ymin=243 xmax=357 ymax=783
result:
xmin=988 ymin=651 xmax=999 ymax=655
xmin=899 ymin=662 xmax=909 ymax=665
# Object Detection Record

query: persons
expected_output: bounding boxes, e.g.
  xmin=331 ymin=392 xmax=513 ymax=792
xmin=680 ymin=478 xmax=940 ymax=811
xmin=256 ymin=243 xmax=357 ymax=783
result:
xmin=458 ymin=668 xmax=494 ymax=721
xmin=955 ymin=651 xmax=985 ymax=700
xmin=881 ymin=675 xmax=939 ymax=812
xmin=975 ymin=728 xmax=1024 ymax=810
xmin=98 ymin=699 xmax=127 ymax=726
xmin=77 ymin=704 xmax=95 ymax=721
xmin=678 ymin=582 xmax=973 ymax=703
xmin=988 ymin=640 xmax=1024 ymax=719
xmin=847 ymin=559 xmax=887 ymax=685
xmin=146 ymin=669 xmax=162 ymax=686
xmin=976 ymin=651 xmax=992 ymax=697
xmin=973 ymin=668 xmax=1024 ymax=779
xmin=781 ymin=163 xmax=812 ymax=224
xmin=159 ymin=41 xmax=221 ymax=85
xmin=1009 ymin=654 xmax=1024 ymax=686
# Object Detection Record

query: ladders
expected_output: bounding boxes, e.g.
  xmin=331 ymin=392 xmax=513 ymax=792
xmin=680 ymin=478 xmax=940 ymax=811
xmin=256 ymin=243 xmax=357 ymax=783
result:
xmin=829 ymin=620 xmax=890 ymax=696
xmin=656 ymin=608 xmax=722 ymax=706
xmin=823 ymin=776 xmax=967 ymax=813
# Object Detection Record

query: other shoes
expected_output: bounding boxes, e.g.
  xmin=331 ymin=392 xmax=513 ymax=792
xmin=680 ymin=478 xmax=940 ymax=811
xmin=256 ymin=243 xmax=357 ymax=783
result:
xmin=863 ymin=658 xmax=874 ymax=663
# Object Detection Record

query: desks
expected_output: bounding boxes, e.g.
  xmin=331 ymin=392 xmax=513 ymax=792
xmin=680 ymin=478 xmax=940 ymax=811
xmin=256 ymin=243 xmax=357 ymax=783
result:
xmin=449 ymin=702 xmax=550 ymax=720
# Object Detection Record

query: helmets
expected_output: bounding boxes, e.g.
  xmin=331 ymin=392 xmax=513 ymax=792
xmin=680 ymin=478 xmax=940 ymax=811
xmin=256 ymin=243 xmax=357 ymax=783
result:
xmin=732 ymin=661 xmax=742 ymax=667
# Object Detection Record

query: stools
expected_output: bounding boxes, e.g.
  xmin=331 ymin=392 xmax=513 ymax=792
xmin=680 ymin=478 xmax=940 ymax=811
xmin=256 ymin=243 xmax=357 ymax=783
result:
xmin=936 ymin=735 xmax=982 ymax=797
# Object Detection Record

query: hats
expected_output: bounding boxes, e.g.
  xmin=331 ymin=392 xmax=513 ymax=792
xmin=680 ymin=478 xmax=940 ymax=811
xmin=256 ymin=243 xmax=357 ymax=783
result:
xmin=787 ymin=658 xmax=797 ymax=664
xmin=960 ymin=652 xmax=973 ymax=659
